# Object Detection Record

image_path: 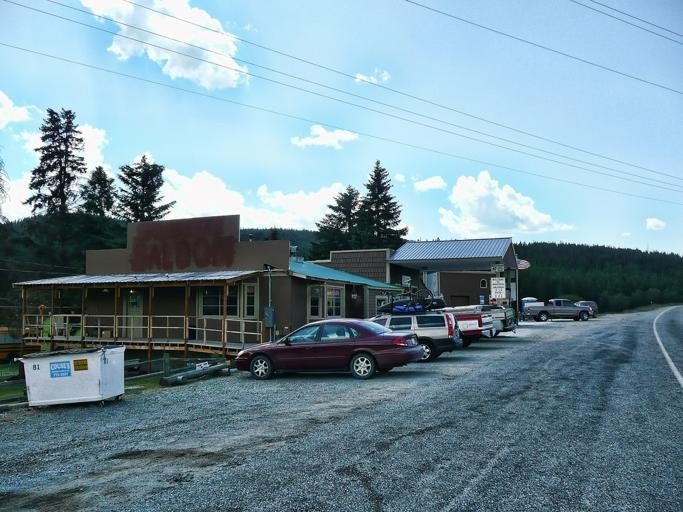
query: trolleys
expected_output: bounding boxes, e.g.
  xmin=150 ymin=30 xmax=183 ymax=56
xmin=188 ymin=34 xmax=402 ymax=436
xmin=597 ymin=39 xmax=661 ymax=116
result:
xmin=15 ymin=342 xmax=131 ymax=415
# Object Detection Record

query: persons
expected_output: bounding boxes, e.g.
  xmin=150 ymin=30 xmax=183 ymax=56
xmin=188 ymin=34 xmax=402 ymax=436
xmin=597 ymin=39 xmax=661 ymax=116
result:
xmin=489 ymin=298 xmax=496 ymax=306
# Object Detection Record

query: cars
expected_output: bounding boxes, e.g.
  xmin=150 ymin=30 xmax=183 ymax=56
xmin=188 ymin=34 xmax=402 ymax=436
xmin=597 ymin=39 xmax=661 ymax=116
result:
xmin=575 ymin=301 xmax=598 ymax=318
xmin=235 ymin=318 xmax=425 ymax=380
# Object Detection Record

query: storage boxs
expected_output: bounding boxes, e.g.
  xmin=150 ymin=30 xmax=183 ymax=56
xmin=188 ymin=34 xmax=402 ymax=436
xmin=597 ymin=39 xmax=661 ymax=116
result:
xmin=101 ymin=329 xmax=112 ymax=338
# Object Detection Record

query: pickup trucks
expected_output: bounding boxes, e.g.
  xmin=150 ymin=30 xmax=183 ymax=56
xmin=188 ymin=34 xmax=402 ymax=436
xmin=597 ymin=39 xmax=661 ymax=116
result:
xmin=430 ymin=303 xmax=518 ymax=349
xmin=524 ymin=299 xmax=594 ymax=323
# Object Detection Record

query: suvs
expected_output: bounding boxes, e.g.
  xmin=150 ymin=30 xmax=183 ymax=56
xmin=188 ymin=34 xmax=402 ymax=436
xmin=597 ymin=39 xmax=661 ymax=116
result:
xmin=359 ymin=311 xmax=464 ymax=362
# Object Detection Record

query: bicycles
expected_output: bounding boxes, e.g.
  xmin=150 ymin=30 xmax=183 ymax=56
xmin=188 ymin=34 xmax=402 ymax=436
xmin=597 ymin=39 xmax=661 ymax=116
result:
xmin=392 ymin=279 xmax=434 ymax=310
